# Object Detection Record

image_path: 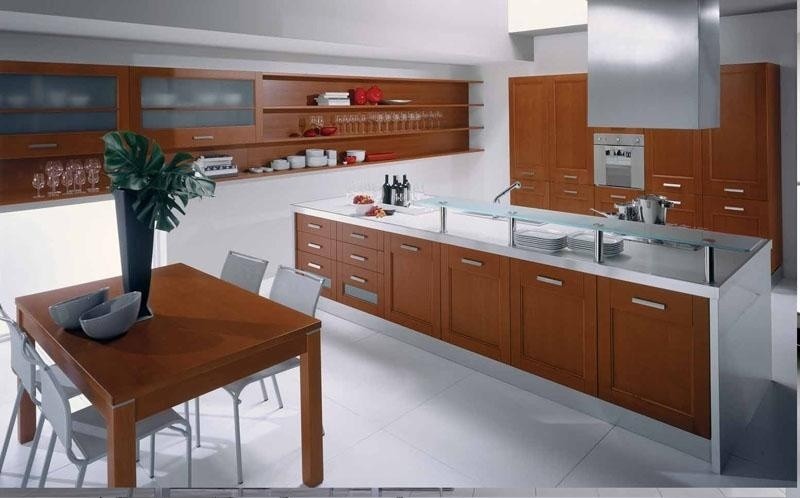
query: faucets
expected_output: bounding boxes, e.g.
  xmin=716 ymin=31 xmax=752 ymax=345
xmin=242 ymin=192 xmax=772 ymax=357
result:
xmin=588 ymin=205 xmax=611 ymax=219
xmin=492 ymin=181 xmax=522 ymax=203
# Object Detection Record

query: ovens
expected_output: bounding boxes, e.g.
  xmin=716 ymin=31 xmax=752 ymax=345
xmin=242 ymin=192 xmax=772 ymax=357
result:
xmin=593 ymin=133 xmax=644 ymax=190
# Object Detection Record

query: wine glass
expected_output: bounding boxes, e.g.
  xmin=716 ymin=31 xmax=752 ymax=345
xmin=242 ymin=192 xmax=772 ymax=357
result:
xmin=335 ymin=110 xmax=443 ymax=136
xmin=311 ymin=115 xmax=325 ymax=137
xmin=31 ymin=158 xmax=101 ymax=199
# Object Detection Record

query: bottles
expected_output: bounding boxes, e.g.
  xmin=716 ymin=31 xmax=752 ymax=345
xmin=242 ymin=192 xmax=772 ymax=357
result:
xmin=382 ymin=174 xmax=410 ymax=208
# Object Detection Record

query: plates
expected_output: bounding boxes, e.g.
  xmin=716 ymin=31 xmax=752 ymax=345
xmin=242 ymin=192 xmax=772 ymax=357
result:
xmin=567 ymin=230 xmax=624 ymax=258
xmin=385 ymin=99 xmax=412 ymax=105
xmin=514 ymin=227 xmax=568 ymax=253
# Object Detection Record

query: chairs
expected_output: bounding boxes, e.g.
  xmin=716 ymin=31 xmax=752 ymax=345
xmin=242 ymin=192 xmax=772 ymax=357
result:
xmin=0 ymin=304 xmax=140 ymax=488
xmin=20 ymin=333 xmax=192 ymax=489
xmin=220 ymin=250 xmax=270 ymax=295
xmin=195 ymin=265 xmax=326 ymax=485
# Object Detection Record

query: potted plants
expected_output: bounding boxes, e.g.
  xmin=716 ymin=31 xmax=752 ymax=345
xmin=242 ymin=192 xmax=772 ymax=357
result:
xmin=101 ymin=130 xmax=217 ymax=319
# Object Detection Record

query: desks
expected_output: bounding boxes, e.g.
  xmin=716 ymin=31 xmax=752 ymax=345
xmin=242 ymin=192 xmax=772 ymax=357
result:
xmin=13 ymin=262 xmax=324 ymax=487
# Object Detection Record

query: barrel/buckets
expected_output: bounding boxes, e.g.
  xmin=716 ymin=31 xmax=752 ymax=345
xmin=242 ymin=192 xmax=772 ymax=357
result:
xmin=635 ymin=194 xmax=665 ymax=225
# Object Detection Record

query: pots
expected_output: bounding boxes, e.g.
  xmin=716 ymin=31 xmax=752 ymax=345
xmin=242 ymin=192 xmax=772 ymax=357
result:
xmin=590 ymin=194 xmax=674 ymax=225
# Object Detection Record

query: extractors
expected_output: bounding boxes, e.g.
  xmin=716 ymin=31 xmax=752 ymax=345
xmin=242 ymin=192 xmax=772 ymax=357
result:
xmin=587 ymin=0 xmax=721 ymax=129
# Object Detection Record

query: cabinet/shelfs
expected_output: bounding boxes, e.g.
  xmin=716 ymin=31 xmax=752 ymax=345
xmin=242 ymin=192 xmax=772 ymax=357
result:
xmin=294 ymin=212 xmax=336 ymax=317
xmin=597 ymin=273 xmax=711 ymax=463
xmin=130 ymin=66 xmax=256 ymax=150
xmin=511 ymin=256 xmax=597 ymax=418
xmin=383 ymin=231 xmax=441 ymax=356
xmin=336 ymin=221 xmax=383 ymax=334
xmin=0 ymin=60 xmax=130 ymax=160
xmin=441 ymin=243 xmax=511 ymax=385
xmin=256 ymin=71 xmax=484 ymax=145
xmin=510 ymin=59 xmax=783 ymax=275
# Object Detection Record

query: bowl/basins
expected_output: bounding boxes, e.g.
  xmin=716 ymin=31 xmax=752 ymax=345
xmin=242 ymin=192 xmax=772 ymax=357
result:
xmin=350 ymin=201 xmax=377 ymax=216
xmin=384 ymin=209 xmax=396 ymax=215
xmin=343 ymin=148 xmax=366 ymax=164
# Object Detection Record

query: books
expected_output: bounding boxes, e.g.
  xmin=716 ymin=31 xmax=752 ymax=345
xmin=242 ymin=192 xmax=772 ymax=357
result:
xmin=196 ymin=153 xmax=239 ymax=178
xmin=313 ymin=92 xmax=350 ymax=106
